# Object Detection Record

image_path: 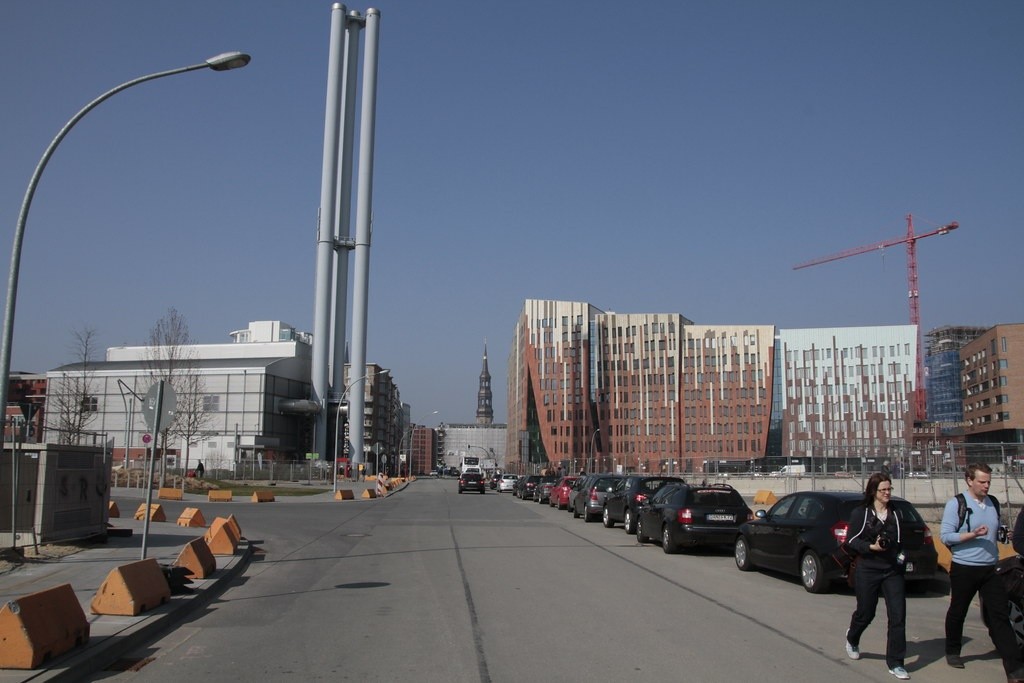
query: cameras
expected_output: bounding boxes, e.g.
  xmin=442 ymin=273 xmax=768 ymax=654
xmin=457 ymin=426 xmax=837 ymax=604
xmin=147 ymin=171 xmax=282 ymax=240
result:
xmin=878 ymin=534 xmax=892 ymax=549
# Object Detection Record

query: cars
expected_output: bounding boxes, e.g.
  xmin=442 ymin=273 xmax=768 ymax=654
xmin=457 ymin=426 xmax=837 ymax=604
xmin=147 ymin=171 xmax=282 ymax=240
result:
xmin=733 ymin=490 xmax=937 ymax=594
xmin=489 ymin=474 xmax=502 ymax=489
xmin=497 ymin=475 xmax=519 ymax=493
xmin=512 ymin=475 xmax=544 ymax=500
xmin=909 ymin=472 xmax=926 ymax=478
xmin=457 ymin=471 xmax=485 ymax=495
xmin=567 ymin=475 xmax=591 ymax=512
xmin=835 ymin=472 xmax=850 ymax=478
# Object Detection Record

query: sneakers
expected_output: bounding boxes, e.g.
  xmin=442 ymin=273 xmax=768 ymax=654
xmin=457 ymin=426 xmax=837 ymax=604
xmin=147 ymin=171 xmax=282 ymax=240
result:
xmin=889 ymin=666 xmax=909 ymax=679
xmin=846 ymin=628 xmax=860 ymax=660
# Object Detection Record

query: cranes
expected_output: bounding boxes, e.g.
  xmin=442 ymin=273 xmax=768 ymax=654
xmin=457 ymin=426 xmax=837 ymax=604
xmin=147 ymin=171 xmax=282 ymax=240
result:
xmin=792 ymin=213 xmax=959 ymax=423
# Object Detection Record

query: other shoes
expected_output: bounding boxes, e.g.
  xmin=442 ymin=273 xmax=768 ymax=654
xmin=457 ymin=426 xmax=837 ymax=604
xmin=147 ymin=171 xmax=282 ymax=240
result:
xmin=945 ymin=652 xmax=964 ymax=668
xmin=1007 ymin=667 xmax=1024 ymax=683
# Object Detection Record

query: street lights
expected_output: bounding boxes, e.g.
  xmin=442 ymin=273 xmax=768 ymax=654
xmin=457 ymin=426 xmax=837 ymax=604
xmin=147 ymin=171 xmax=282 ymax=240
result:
xmin=409 ymin=411 xmax=438 ymax=476
xmin=398 ymin=429 xmax=420 ymax=478
xmin=334 ymin=369 xmax=390 ymax=492
xmin=1 ymin=52 xmax=252 ymax=443
xmin=590 ymin=429 xmax=600 ymax=474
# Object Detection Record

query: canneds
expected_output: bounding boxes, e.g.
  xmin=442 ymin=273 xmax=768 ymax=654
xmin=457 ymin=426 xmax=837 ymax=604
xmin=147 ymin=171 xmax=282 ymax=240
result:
xmin=1000 ymin=524 xmax=1009 ymax=545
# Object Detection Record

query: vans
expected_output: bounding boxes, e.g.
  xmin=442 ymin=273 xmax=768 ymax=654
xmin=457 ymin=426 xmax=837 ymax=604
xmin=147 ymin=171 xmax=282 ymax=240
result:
xmin=778 ymin=465 xmax=805 ymax=477
xmin=464 ymin=467 xmax=480 ymax=474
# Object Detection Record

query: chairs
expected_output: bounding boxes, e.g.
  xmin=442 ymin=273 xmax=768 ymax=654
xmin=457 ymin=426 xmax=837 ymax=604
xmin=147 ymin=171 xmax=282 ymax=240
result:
xmin=806 ymin=502 xmax=822 ymax=519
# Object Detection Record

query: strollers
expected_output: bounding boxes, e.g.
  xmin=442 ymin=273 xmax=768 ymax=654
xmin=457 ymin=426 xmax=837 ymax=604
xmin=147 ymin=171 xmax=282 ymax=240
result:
xmin=188 ymin=470 xmax=197 ymax=477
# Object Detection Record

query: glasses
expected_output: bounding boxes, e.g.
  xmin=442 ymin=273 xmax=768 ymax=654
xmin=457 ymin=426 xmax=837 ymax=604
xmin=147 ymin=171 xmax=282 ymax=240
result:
xmin=876 ymin=487 xmax=894 ymax=492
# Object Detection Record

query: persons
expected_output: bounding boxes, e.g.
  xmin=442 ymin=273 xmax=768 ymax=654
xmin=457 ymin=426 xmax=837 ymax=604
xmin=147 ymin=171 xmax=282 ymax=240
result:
xmin=845 ymin=472 xmax=914 ymax=679
xmin=195 ymin=460 xmax=204 ymax=478
xmin=1012 ymin=505 xmax=1024 ymax=555
xmin=939 ymin=461 xmax=1024 ymax=683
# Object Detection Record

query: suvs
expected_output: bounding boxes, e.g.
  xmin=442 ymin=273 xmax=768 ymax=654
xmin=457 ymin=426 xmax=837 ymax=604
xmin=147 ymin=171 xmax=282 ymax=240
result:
xmin=574 ymin=473 xmax=626 ymax=523
xmin=636 ymin=483 xmax=755 ymax=554
xmin=533 ymin=475 xmax=558 ymax=504
xmin=603 ymin=474 xmax=687 ymax=534
xmin=451 ymin=470 xmax=460 ymax=477
xmin=549 ymin=476 xmax=580 ymax=511
xmin=430 ymin=470 xmax=438 ymax=477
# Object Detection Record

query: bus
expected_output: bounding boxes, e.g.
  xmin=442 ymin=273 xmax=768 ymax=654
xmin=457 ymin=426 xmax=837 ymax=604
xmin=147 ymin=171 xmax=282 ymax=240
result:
xmin=462 ymin=456 xmax=482 ymax=473
